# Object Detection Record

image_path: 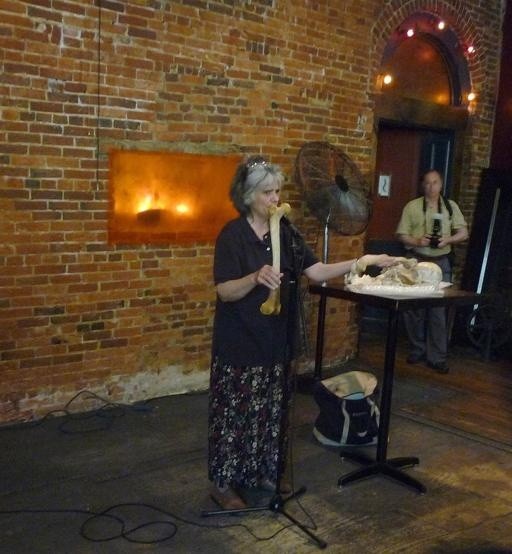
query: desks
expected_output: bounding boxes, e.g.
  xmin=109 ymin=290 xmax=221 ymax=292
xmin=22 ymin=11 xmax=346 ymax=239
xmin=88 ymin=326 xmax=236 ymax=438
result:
xmin=308 ymin=282 xmax=482 ymax=495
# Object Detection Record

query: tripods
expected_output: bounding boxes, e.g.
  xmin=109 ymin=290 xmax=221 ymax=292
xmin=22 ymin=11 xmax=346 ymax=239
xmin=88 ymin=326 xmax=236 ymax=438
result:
xmin=201 ymin=233 xmax=327 ymax=548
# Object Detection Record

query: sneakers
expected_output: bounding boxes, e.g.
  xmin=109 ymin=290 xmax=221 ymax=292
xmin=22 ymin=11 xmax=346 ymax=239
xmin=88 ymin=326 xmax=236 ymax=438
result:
xmin=429 ymin=359 xmax=450 ymax=373
xmin=406 ymin=353 xmax=423 ymax=364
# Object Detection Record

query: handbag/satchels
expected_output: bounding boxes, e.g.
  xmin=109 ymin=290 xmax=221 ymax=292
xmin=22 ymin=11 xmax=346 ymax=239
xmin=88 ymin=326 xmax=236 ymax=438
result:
xmin=312 ymin=370 xmax=381 ymax=448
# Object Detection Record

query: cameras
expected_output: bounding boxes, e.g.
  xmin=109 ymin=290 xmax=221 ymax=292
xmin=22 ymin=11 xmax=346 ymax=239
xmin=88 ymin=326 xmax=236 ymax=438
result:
xmin=426 ymin=214 xmax=442 ymax=248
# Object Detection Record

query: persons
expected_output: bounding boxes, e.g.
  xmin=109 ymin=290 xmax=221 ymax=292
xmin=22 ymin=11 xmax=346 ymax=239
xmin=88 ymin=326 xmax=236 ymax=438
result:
xmin=393 ymin=169 xmax=469 ymax=374
xmin=204 ymin=156 xmax=402 ymax=520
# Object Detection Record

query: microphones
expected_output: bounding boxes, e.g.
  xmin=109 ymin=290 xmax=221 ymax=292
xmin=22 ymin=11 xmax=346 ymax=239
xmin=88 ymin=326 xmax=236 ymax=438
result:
xmin=269 ymin=206 xmax=298 ymax=233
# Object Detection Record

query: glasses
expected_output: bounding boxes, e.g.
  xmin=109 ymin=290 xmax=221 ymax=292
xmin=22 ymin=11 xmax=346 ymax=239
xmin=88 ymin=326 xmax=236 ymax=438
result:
xmin=240 ymin=159 xmax=270 ymax=192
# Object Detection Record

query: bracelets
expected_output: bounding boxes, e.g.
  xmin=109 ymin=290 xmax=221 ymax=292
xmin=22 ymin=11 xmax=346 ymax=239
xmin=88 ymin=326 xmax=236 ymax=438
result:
xmin=355 ymin=256 xmax=360 ymax=264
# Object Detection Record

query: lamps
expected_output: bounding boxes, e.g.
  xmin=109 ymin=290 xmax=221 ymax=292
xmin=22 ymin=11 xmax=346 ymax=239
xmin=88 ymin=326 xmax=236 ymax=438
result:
xmin=374 ymin=70 xmax=393 ymax=85
xmin=428 ymin=18 xmax=446 ymax=30
xmin=398 ymin=27 xmax=414 ymax=39
xmin=459 ymin=44 xmax=475 ymax=53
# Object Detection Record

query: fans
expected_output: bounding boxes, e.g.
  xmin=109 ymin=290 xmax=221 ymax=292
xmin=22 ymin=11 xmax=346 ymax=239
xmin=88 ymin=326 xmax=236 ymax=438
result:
xmin=297 ymin=142 xmax=374 ymax=395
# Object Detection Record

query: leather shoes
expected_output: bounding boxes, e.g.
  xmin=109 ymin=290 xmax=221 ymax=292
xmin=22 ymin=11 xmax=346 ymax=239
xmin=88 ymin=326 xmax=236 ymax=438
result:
xmin=262 ymin=477 xmax=293 ymax=494
xmin=209 ymin=488 xmax=249 ymax=518
